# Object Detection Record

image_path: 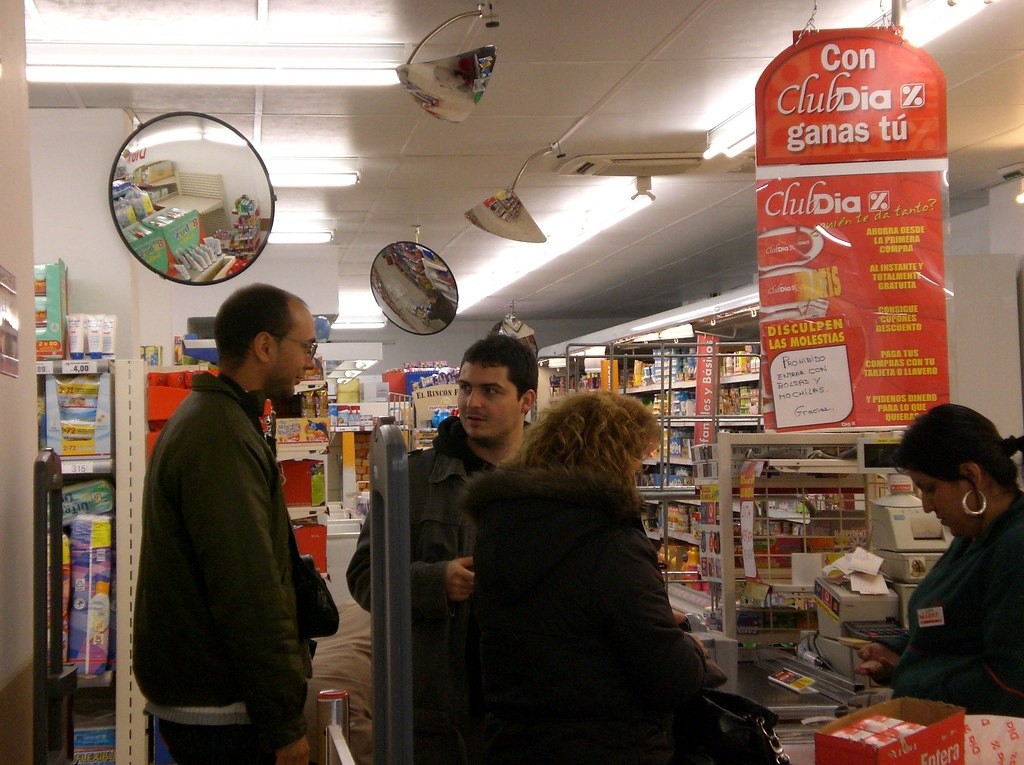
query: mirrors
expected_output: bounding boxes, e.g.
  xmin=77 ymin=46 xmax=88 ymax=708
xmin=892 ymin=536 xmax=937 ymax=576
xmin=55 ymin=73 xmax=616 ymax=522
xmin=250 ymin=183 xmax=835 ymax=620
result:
xmin=370 ymin=240 xmax=459 ymax=336
xmin=107 ymin=111 xmax=275 ymax=287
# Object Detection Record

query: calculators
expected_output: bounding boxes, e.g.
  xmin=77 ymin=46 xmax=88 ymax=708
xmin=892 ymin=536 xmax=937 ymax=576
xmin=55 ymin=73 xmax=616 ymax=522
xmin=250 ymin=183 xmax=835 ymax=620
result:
xmin=843 ymin=619 xmax=909 ymax=644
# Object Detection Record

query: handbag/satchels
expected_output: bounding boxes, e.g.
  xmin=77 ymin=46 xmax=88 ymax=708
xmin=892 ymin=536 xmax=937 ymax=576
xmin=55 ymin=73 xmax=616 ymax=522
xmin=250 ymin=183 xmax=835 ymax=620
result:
xmin=671 ymin=689 xmax=792 ymax=765
xmin=290 ymin=554 xmax=339 ymax=639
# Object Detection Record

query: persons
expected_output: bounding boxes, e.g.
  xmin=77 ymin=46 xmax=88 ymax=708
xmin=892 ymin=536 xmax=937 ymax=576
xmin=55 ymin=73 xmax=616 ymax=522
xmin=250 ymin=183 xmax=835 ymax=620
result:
xmin=345 ymin=337 xmax=541 ymax=765
xmin=131 ymin=284 xmax=316 ymax=765
xmin=425 ymin=288 xmax=456 ymax=326
xmin=854 ymin=404 xmax=1024 ymax=720
xmin=459 ymin=391 xmax=709 ymax=765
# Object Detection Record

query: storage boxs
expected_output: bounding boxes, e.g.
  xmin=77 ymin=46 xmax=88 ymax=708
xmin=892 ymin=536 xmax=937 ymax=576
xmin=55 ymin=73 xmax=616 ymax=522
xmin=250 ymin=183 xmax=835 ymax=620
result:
xmin=158 ymin=186 xmax=169 ymax=197
xmin=136 ymin=159 xmax=173 ymax=183
xmin=174 ymin=334 xmax=199 ymax=366
xmin=548 ymin=347 xmax=833 ymax=607
xmin=145 ymin=346 xmax=162 ymax=366
xmin=814 ymin=695 xmax=966 ymax=765
xmin=46 ymin=374 xmax=112 ymax=460
xmin=146 ymin=188 xmax=161 ymax=204
xmin=34 ymin=256 xmax=68 ymax=361
xmin=140 ymin=205 xmax=206 ymax=265
xmin=121 ymin=222 xmax=179 ymax=276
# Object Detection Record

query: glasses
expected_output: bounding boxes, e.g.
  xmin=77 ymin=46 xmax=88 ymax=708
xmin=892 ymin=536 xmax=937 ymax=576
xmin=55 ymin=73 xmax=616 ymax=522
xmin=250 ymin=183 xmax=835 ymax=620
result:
xmin=269 ymin=332 xmax=319 ymax=359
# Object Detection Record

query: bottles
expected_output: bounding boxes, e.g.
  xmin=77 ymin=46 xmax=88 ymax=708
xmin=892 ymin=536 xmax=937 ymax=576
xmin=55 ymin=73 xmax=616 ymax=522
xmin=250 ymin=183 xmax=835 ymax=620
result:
xmin=619 ymin=359 xmax=654 ymax=388
xmin=668 ymin=503 xmax=700 ymax=539
xmin=657 ymin=541 xmax=712 ymax=594
xmin=720 ymin=351 xmax=760 ymax=376
xmin=687 ymin=347 xmax=697 ymax=381
xmin=586 ymin=369 xmax=601 ymax=389
xmin=553 ymin=377 xmax=565 ymax=396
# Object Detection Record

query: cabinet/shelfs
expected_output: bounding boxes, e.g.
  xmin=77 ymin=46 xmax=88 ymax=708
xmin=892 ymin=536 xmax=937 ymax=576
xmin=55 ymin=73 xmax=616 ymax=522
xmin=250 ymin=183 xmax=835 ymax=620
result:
xmin=535 ymin=285 xmax=855 ymax=613
xmin=36 ymin=358 xmax=150 ymax=765
xmin=421 ymin=258 xmax=458 ymax=304
xmin=231 ymin=203 xmax=261 ymax=258
